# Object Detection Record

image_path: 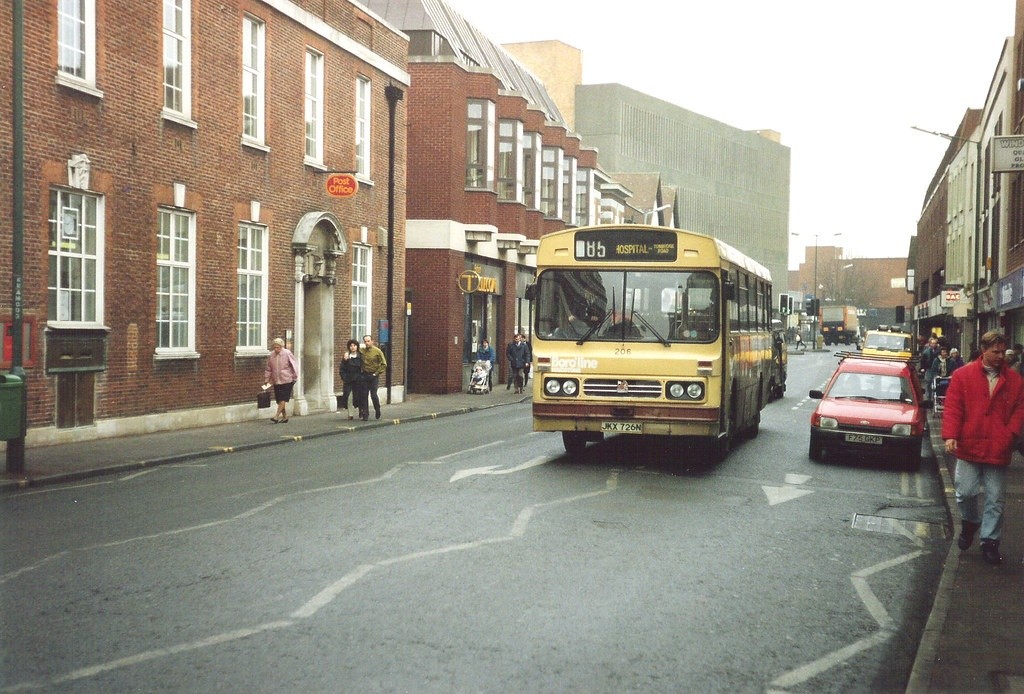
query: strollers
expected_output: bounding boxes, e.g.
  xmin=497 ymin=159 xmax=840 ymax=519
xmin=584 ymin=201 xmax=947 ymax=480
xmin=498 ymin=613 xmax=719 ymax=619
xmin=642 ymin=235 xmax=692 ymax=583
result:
xmin=467 ymin=361 xmax=492 ymax=395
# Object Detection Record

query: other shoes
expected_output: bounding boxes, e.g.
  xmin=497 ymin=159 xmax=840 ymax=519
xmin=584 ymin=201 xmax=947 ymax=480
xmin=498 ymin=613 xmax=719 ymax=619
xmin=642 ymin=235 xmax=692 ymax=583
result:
xmin=359 ymin=411 xmax=363 ymax=418
xmin=363 ymin=415 xmax=368 ymax=420
xmin=271 ymin=418 xmax=279 ymax=424
xmin=958 ymin=521 xmax=981 ymax=551
xmin=981 ymin=539 xmax=1004 ymax=563
xmin=376 ymin=412 xmax=381 ymax=419
xmin=279 ymin=419 xmax=288 ymax=423
xmin=348 ymin=416 xmax=353 ymax=420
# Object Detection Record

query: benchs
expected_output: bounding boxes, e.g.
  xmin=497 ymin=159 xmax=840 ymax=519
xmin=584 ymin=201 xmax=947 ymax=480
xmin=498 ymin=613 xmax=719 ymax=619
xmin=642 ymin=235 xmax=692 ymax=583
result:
xmin=626 ymin=308 xmax=715 ymax=337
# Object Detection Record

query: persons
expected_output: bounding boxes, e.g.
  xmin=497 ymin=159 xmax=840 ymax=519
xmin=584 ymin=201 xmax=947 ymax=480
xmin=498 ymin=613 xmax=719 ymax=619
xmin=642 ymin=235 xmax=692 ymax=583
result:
xmin=339 ymin=339 xmax=364 ymax=420
xmin=264 ymin=338 xmax=298 ymax=424
xmin=520 ymin=335 xmax=533 ymax=387
xmin=507 ymin=340 xmax=515 ymax=389
xmin=568 ymin=290 xmax=605 ymax=330
xmin=471 ymin=367 xmax=484 ymax=386
xmin=344 ymin=335 xmax=387 ymax=421
xmin=476 ymin=340 xmax=495 ymax=391
xmin=506 ymin=334 xmax=530 ymax=394
xmin=942 ymin=330 xmax=1024 ymax=564
xmin=919 ymin=333 xmax=1024 ymax=402
xmin=795 ymin=327 xmax=807 ymax=349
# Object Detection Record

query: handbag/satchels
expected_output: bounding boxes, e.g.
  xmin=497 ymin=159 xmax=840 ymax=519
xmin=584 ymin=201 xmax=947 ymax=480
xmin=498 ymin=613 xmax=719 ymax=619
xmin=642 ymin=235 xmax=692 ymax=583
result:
xmin=257 ymin=392 xmax=271 ymax=409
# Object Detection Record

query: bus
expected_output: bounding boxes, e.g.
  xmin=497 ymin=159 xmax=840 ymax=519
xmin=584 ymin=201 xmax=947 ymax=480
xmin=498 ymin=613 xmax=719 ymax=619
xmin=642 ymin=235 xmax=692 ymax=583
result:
xmin=525 ymin=223 xmax=773 ymax=461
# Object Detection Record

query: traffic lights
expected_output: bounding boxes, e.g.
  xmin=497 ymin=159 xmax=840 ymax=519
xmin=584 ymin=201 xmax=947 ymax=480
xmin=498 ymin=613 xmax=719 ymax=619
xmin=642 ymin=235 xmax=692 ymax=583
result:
xmin=789 ymin=297 xmax=794 ymax=315
xmin=806 ymin=300 xmax=813 ymax=316
xmin=779 ymin=294 xmax=788 ymax=314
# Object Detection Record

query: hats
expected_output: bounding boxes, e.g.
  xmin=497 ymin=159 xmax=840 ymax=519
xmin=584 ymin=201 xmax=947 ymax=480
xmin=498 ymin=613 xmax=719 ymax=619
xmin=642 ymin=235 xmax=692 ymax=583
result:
xmin=1006 ymin=349 xmax=1014 ymax=355
xmin=272 ymin=338 xmax=285 ymax=348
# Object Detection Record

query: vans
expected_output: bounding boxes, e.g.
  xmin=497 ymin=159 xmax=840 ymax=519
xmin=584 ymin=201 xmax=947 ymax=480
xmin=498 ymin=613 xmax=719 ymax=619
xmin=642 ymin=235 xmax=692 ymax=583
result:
xmin=856 ymin=328 xmax=914 ymax=364
xmin=769 ymin=319 xmax=787 ymax=402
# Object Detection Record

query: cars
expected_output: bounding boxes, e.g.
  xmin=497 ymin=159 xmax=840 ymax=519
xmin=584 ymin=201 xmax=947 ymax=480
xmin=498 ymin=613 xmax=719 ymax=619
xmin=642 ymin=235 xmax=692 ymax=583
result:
xmin=810 ymin=357 xmax=935 ymax=469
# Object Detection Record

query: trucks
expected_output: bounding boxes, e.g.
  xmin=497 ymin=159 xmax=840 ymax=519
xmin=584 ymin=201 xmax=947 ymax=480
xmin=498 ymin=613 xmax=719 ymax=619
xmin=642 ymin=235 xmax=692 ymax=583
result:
xmin=819 ymin=306 xmax=860 ymax=345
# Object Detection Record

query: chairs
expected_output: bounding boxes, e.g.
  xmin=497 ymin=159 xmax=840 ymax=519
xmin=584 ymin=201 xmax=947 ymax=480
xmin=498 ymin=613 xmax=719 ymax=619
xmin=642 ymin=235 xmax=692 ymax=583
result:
xmin=842 ymin=377 xmax=907 ymax=400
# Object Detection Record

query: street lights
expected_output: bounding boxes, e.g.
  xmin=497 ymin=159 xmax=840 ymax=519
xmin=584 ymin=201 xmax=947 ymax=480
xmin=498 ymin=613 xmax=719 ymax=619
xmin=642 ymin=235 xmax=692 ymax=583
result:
xmin=791 ymin=232 xmax=842 ymax=350
